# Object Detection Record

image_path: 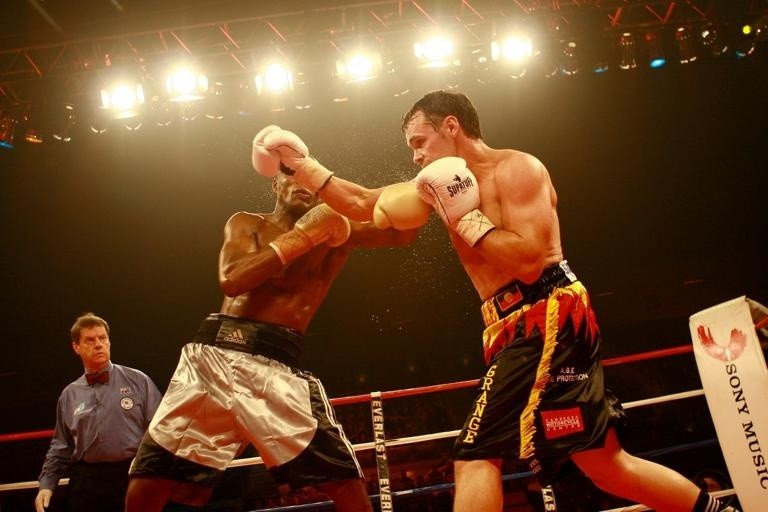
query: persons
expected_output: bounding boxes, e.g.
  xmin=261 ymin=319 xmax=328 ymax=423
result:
xmin=249 ymin=90 xmax=742 ymax=511
xmin=34 ymin=312 xmax=165 ymax=512
xmin=693 ymin=464 xmax=733 ymax=504
xmin=123 ymin=157 xmax=429 ymax=508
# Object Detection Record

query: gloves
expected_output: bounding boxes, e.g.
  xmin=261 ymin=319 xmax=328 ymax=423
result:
xmin=249 ymin=125 xmax=333 ymax=196
xmin=267 ymin=203 xmax=351 ymax=264
xmin=416 ymin=156 xmax=495 ymax=247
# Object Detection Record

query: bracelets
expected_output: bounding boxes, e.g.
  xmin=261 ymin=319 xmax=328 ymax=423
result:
xmin=453 ymin=210 xmax=499 ymax=249
xmin=264 ymin=231 xmax=310 ymax=268
xmin=294 ymin=161 xmax=338 ymax=200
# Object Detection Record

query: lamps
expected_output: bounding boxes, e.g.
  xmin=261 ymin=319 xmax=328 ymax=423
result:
xmin=96 ymin=20 xmax=534 ymax=119
xmin=0 ymin=0 xmax=768 ymax=147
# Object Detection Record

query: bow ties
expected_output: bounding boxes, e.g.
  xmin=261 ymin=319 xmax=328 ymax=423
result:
xmin=85 ymin=372 xmax=110 ymax=385
